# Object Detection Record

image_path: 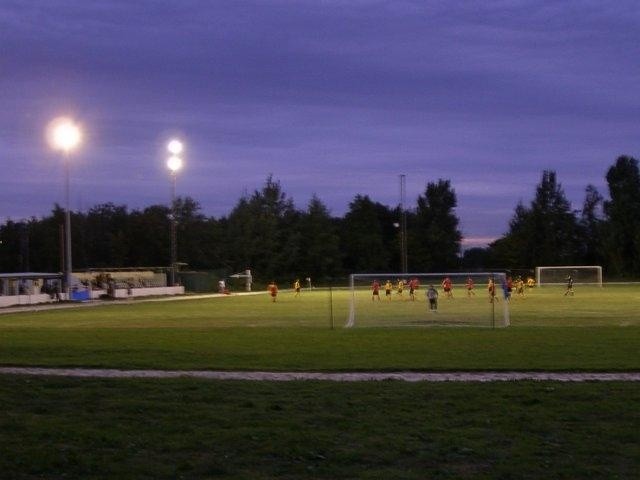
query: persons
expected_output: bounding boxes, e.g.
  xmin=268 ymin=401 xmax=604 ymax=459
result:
xmin=293 ymin=279 xmax=302 ymax=298
xmin=272 ymin=283 xmax=279 ymax=304
xmin=19 ymin=278 xmax=28 ymax=294
xmin=370 ymin=275 xmax=537 ymax=311
xmin=564 ymin=272 xmax=575 ymax=296
xmin=267 ymin=278 xmax=275 ymax=301
xmin=219 ymin=278 xmax=225 ymax=293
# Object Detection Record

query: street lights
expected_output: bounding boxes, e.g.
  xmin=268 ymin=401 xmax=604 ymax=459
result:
xmin=48 ymin=120 xmax=80 ymax=289
xmin=168 ymin=139 xmax=182 ymax=284
